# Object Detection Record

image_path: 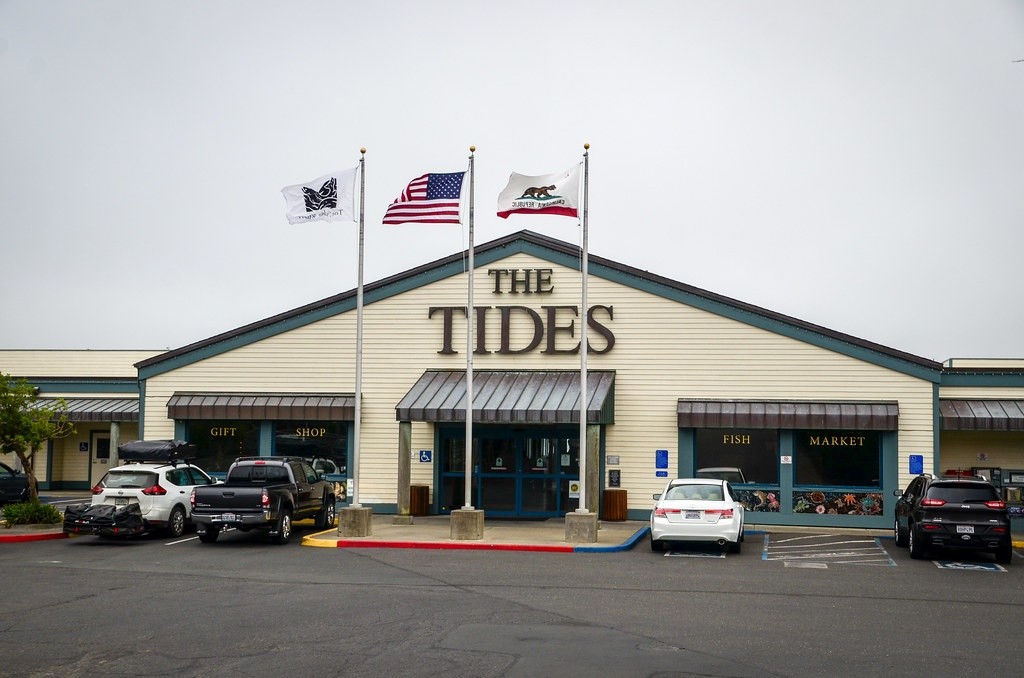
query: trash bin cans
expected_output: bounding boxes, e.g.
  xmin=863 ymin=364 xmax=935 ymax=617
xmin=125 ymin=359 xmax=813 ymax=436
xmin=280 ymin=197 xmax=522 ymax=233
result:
xmin=601 ymin=489 xmax=627 ymax=522
xmin=410 ymin=485 xmax=429 ymax=517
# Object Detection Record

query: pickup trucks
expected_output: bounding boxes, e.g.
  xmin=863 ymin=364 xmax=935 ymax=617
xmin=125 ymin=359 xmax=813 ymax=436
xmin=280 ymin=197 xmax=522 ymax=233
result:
xmin=189 ymin=455 xmax=336 ymax=546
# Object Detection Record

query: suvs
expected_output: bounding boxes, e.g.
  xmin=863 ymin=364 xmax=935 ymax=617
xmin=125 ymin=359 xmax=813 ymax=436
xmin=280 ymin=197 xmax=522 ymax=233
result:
xmin=91 ymin=459 xmax=225 ymax=538
xmin=892 ymin=472 xmax=1013 ymax=564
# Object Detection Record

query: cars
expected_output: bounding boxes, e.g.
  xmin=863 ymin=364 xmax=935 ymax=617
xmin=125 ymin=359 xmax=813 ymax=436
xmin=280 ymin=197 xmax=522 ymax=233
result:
xmin=696 ymin=466 xmax=756 ymax=485
xmin=303 ymin=457 xmax=340 ymax=474
xmin=649 ymin=478 xmax=747 ymax=554
xmin=0 ymin=460 xmax=39 ymax=504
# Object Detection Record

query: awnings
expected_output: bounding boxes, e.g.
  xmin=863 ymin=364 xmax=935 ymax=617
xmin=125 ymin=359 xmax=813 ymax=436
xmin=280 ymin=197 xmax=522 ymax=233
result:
xmin=165 ymin=396 xmax=356 ymax=420
xmin=939 ymin=400 xmax=1024 ymax=431
xmin=0 ymin=399 xmax=139 ymax=421
xmin=677 ymin=402 xmax=898 ymax=430
xmin=394 ymin=372 xmax=614 ymax=424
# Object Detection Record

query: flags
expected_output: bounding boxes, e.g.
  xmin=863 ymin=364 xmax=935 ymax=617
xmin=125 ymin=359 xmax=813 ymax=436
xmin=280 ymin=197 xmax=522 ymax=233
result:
xmin=382 ymin=171 xmax=466 ymax=224
xmin=280 ymin=167 xmax=357 ymax=224
xmin=497 ymin=164 xmax=581 ymax=218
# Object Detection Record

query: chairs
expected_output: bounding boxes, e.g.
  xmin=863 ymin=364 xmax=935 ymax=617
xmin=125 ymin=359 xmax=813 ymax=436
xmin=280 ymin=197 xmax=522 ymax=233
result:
xmin=670 ymin=493 xmax=720 ymax=500
